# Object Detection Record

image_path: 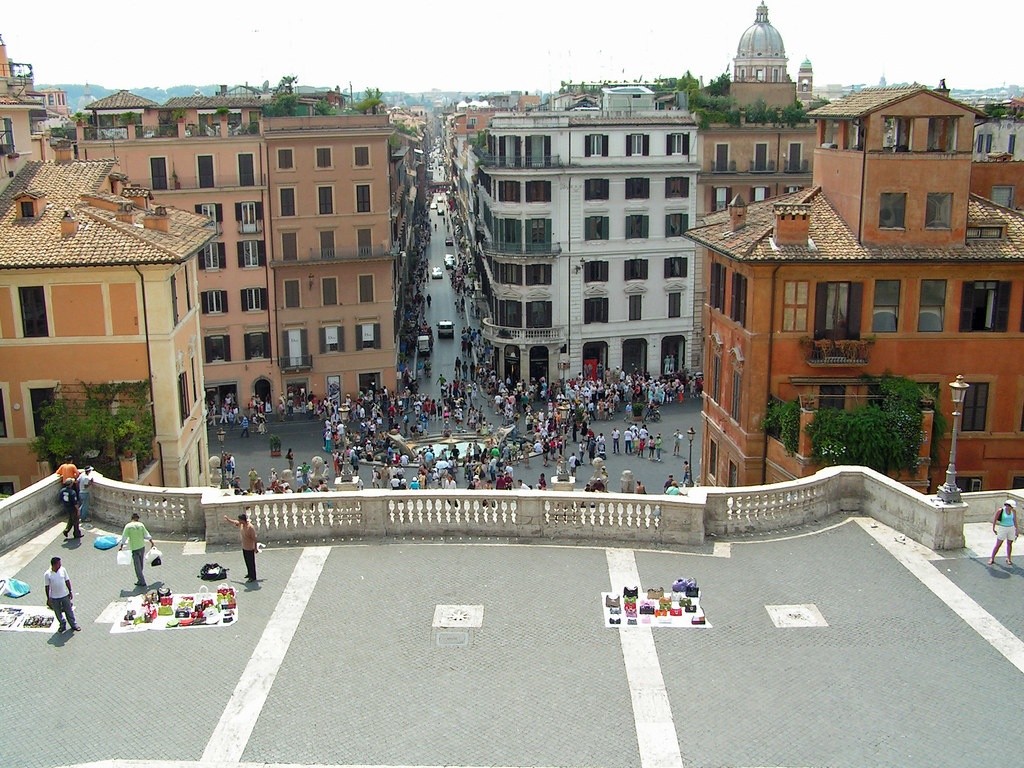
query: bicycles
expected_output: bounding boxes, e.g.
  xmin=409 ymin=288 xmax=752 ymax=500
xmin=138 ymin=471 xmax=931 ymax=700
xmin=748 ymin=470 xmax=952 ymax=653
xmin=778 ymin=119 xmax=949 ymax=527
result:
xmin=643 ymin=403 xmax=660 ymax=422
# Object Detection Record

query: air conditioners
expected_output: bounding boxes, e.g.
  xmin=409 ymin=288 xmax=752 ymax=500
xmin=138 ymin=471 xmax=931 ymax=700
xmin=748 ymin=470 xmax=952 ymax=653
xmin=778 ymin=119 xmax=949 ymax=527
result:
xmin=970 ymin=479 xmax=981 ymax=491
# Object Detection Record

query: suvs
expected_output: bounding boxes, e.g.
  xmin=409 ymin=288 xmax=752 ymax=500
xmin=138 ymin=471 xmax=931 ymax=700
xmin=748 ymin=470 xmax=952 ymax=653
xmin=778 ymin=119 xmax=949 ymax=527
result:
xmin=436 ymin=319 xmax=455 ymax=339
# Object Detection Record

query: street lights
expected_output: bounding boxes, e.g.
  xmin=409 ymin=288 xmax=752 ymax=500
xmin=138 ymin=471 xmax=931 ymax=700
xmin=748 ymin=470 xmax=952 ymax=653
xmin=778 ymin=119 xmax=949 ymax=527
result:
xmin=216 ymin=428 xmax=229 ymax=489
xmin=336 ymin=403 xmax=352 ymax=482
xmin=555 ymin=400 xmax=570 ymax=482
xmin=937 ymin=374 xmax=970 ymax=503
xmin=684 ymin=426 xmax=696 ymax=487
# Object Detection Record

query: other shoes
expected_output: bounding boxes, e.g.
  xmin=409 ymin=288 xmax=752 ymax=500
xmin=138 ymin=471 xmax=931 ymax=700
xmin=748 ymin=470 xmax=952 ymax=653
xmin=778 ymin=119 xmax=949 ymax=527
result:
xmin=1005 ymin=558 xmax=1012 ymax=565
xmin=244 ymin=574 xmax=248 ymax=578
xmin=246 ymin=578 xmax=253 ymax=583
xmin=81 ymin=534 xmax=84 ymax=537
xmin=988 ymin=559 xmax=994 ymax=564
xmin=63 ymin=531 xmax=68 ymax=538
xmin=59 ymin=627 xmax=66 ymax=632
xmin=76 ymin=627 xmax=81 ymax=631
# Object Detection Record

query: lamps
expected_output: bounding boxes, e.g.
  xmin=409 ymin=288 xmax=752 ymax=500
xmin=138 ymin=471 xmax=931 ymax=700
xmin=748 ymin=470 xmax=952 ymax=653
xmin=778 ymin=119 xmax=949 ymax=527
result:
xmin=575 ymin=257 xmax=585 ymax=274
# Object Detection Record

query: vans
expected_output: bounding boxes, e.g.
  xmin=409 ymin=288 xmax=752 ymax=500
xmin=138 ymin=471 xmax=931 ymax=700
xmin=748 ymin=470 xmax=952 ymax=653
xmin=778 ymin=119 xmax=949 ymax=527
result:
xmin=417 ymin=334 xmax=431 ymax=358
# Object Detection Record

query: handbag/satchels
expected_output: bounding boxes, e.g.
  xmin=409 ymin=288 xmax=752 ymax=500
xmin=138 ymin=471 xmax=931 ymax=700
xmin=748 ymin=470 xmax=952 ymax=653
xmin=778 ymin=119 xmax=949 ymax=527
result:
xmin=119 ymin=582 xmax=237 ymax=628
xmin=997 ymin=508 xmax=1004 ymax=521
xmin=151 ymin=556 xmax=162 ymax=566
xmin=606 ymin=577 xmax=706 ymax=625
xmin=145 ymin=547 xmax=162 ymax=564
xmin=197 ymin=563 xmax=230 ymax=582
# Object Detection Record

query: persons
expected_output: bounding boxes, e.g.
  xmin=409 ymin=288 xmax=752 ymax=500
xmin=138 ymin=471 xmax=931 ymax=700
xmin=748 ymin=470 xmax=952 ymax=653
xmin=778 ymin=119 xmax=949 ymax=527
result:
xmin=59 ymin=478 xmax=84 ymax=538
xmin=398 ymin=139 xmax=484 ymax=357
xmin=44 ymin=557 xmax=81 ymax=632
xmin=77 ymin=465 xmax=94 ymax=522
xmin=119 ymin=513 xmax=155 ymax=587
xmin=988 ymin=499 xmax=1020 ymax=565
xmin=205 ymin=357 xmax=703 ymax=496
xmin=56 ymin=455 xmax=81 ymax=501
xmin=225 ymin=514 xmax=258 ymax=583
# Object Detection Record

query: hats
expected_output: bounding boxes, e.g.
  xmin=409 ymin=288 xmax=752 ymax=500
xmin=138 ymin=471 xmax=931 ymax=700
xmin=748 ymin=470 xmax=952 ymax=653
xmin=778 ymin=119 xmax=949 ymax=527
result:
xmin=597 ymin=478 xmax=601 ymax=481
xmin=84 ymin=465 xmax=94 ymax=471
xmin=65 ymin=477 xmax=75 ymax=485
xmin=1004 ymin=500 xmax=1017 ymax=508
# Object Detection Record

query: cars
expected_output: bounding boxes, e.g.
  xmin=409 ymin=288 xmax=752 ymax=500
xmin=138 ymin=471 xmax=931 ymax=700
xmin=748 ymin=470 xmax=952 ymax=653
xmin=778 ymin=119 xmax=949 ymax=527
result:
xmin=436 ymin=208 xmax=445 ymax=216
xmin=436 ymin=197 xmax=444 ymax=203
xmin=431 ymin=267 xmax=444 ymax=279
xmin=430 ymin=202 xmax=438 ymax=210
xmin=445 ymin=236 xmax=453 ymax=246
xmin=444 ymin=253 xmax=456 ymax=270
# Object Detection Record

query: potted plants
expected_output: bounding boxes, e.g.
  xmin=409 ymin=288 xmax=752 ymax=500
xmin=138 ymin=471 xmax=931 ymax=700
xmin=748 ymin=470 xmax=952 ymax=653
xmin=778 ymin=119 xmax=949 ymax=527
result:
xmin=918 ymin=383 xmax=938 ymax=412
xmin=632 ymin=403 xmax=645 ymax=422
xmin=71 ymin=112 xmax=89 ymax=126
xmin=805 ymin=392 xmax=814 ymax=410
xmin=29 ymin=379 xmax=153 ymax=478
xmin=270 ymin=433 xmax=281 ymax=456
xmin=172 ymin=108 xmax=189 ymax=123
xmin=575 ymin=408 xmax=585 ymax=428
xmin=119 ymin=111 xmax=140 ymax=125
xmin=213 ymin=106 xmax=231 ymax=121
